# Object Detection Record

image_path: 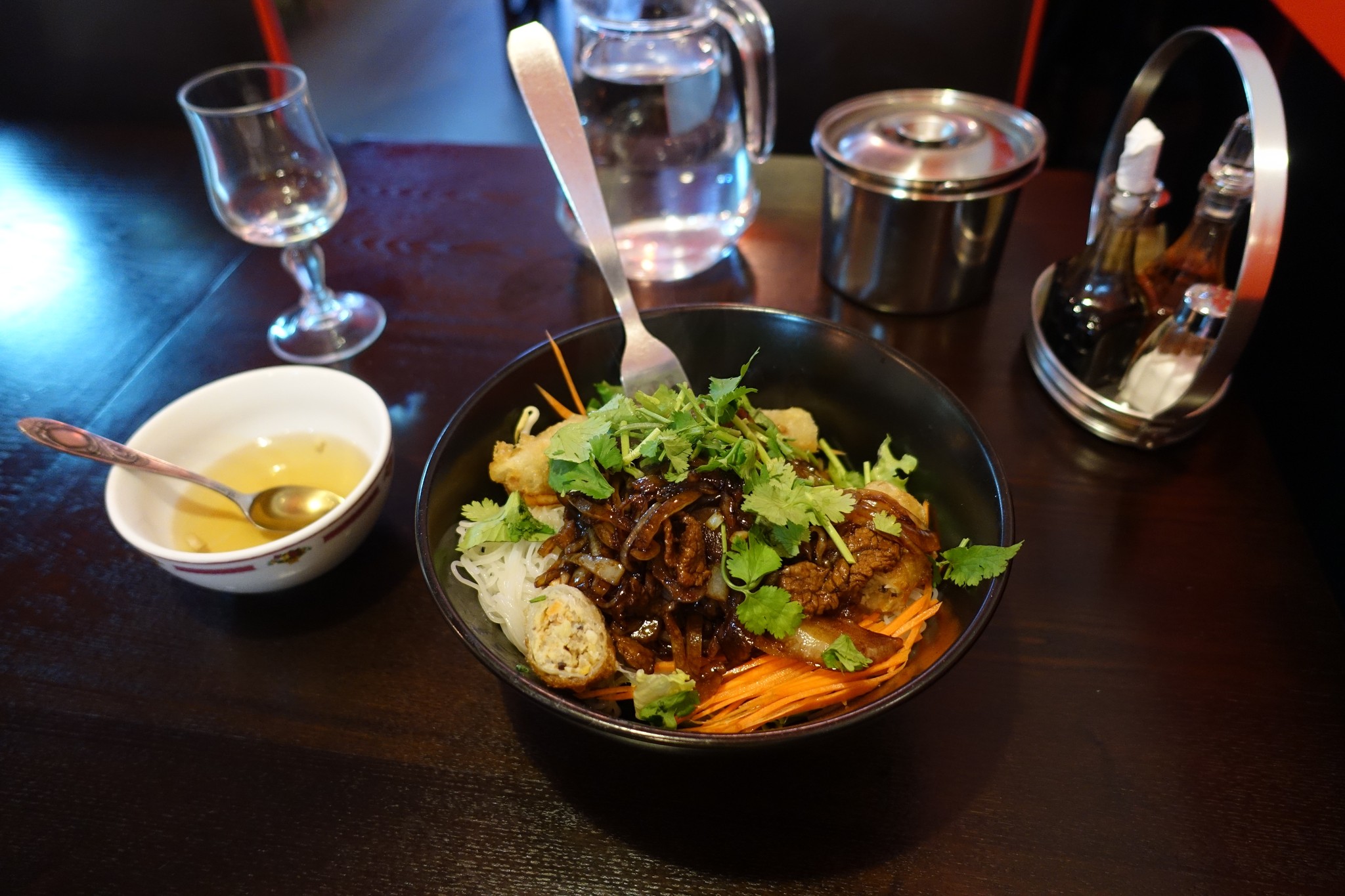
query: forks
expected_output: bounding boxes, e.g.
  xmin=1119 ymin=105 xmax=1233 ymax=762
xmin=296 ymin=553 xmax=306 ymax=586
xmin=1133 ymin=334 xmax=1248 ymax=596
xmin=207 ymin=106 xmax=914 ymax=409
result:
xmin=506 ymin=20 xmax=697 ymax=392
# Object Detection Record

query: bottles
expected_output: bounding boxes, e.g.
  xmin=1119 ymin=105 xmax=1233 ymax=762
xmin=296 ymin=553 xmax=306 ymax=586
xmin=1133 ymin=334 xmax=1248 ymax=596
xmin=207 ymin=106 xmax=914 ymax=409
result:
xmin=1139 ymin=114 xmax=1259 ymax=320
xmin=1047 ymin=171 xmax=1166 ymax=387
xmin=1120 ymin=280 xmax=1235 ymax=417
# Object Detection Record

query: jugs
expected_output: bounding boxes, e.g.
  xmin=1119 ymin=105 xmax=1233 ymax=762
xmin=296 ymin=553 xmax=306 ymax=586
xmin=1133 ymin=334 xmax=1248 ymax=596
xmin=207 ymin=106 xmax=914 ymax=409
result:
xmin=553 ymin=0 xmax=780 ymax=284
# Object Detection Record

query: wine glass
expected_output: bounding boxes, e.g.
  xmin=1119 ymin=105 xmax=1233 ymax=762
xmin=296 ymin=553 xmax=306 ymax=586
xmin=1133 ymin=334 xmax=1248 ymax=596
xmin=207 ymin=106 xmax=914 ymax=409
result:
xmin=179 ymin=62 xmax=386 ymax=364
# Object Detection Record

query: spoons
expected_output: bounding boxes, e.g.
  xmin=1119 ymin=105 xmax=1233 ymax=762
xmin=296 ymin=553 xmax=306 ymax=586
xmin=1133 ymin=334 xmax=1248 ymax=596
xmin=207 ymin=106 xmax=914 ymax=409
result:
xmin=19 ymin=417 xmax=345 ymax=528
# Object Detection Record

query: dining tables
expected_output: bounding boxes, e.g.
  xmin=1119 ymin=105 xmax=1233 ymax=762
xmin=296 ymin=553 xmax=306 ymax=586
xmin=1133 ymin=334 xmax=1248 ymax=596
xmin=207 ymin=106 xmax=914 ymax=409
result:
xmin=0 ymin=119 xmax=1345 ymax=896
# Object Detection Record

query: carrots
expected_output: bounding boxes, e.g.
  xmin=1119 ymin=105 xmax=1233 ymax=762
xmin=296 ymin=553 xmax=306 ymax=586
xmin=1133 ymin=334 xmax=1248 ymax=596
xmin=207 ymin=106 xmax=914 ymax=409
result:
xmin=578 ymin=577 xmax=946 ymax=735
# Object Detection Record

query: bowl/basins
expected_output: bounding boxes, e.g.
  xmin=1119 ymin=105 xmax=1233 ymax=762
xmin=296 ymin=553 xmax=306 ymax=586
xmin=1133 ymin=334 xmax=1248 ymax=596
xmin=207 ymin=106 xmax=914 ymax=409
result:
xmin=417 ymin=304 xmax=1016 ymax=751
xmin=109 ymin=364 xmax=395 ymax=593
xmin=815 ymin=92 xmax=1045 ymax=326
